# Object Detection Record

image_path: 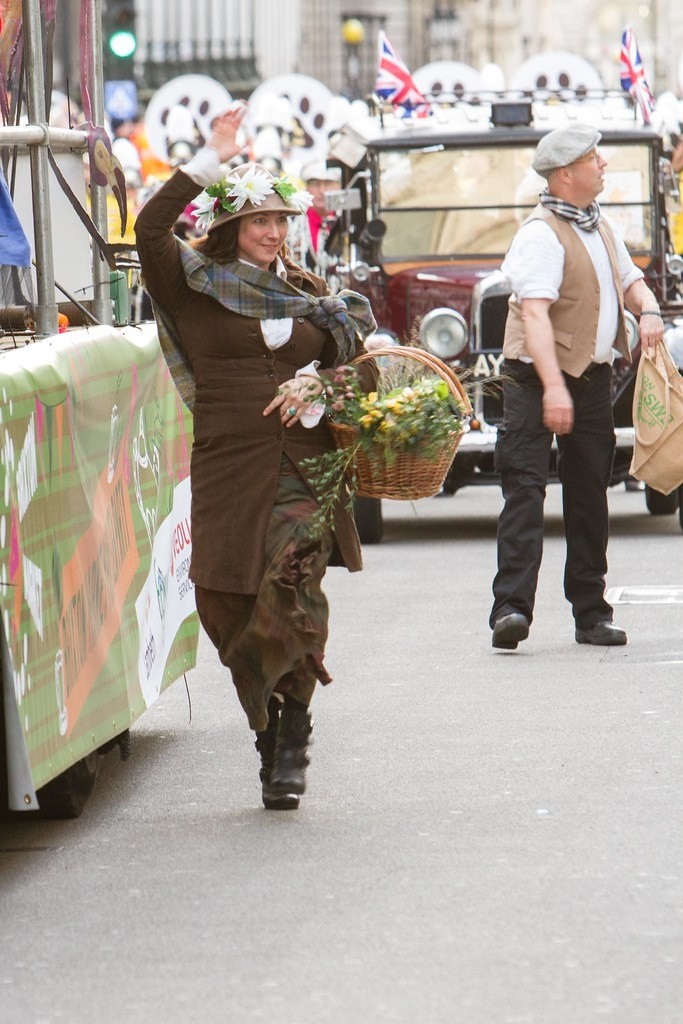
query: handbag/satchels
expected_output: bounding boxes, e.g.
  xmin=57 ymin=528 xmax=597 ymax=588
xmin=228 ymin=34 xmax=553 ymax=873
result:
xmin=628 ymin=339 xmax=683 ymax=495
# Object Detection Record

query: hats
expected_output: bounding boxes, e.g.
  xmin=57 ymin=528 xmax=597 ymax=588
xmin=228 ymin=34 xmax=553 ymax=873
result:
xmin=301 ymin=158 xmax=340 ymax=183
xmin=532 ymin=123 xmax=602 ymax=178
xmin=207 ymin=161 xmax=301 ymax=236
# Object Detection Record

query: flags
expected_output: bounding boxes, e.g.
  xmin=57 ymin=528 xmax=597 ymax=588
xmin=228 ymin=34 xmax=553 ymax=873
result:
xmin=374 ymin=31 xmax=433 ymax=119
xmin=619 ymin=25 xmax=656 ymax=125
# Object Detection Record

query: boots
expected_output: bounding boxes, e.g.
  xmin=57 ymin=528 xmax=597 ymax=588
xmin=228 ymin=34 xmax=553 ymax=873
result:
xmin=270 ymin=711 xmax=313 ymax=793
xmin=254 ymin=734 xmax=300 ymax=808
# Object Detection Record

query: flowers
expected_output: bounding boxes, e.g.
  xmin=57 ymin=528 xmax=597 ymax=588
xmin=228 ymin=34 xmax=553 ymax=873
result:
xmin=187 ymin=164 xmax=316 ymax=232
xmin=277 ymin=364 xmax=477 ymax=533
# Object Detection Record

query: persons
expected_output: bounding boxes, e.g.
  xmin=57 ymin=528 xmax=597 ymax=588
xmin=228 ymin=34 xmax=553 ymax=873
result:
xmin=106 ymin=115 xmax=142 ymax=203
xmin=488 ymin=121 xmax=664 ymax=649
xmin=299 ymin=158 xmax=350 ymax=292
xmin=131 ymin=105 xmax=381 ymax=811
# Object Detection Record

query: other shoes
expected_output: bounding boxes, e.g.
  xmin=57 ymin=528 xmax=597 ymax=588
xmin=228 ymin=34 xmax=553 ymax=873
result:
xmin=492 ymin=613 xmax=529 ymax=649
xmin=575 ymin=621 xmax=627 ymax=646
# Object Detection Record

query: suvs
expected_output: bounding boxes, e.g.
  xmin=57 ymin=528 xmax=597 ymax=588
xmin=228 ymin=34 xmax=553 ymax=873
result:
xmin=326 ymin=103 xmax=682 ymax=541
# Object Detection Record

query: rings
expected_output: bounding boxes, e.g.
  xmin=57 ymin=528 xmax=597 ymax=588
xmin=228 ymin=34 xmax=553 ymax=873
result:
xmin=288 ymin=407 xmax=297 ymax=416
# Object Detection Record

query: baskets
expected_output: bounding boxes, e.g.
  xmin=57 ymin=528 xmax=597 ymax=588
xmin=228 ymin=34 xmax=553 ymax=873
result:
xmin=325 ymin=347 xmax=472 ymax=501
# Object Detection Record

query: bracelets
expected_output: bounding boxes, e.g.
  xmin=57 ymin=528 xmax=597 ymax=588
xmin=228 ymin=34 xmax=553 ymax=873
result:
xmin=641 ymin=311 xmax=661 ymax=317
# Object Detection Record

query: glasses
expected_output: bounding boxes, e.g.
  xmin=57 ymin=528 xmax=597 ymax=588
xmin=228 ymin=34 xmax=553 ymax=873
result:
xmin=570 ymin=150 xmax=600 ymax=165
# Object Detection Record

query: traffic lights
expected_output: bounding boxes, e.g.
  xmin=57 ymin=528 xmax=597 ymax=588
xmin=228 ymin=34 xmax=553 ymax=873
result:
xmin=103 ymin=1 xmax=140 ymax=123
